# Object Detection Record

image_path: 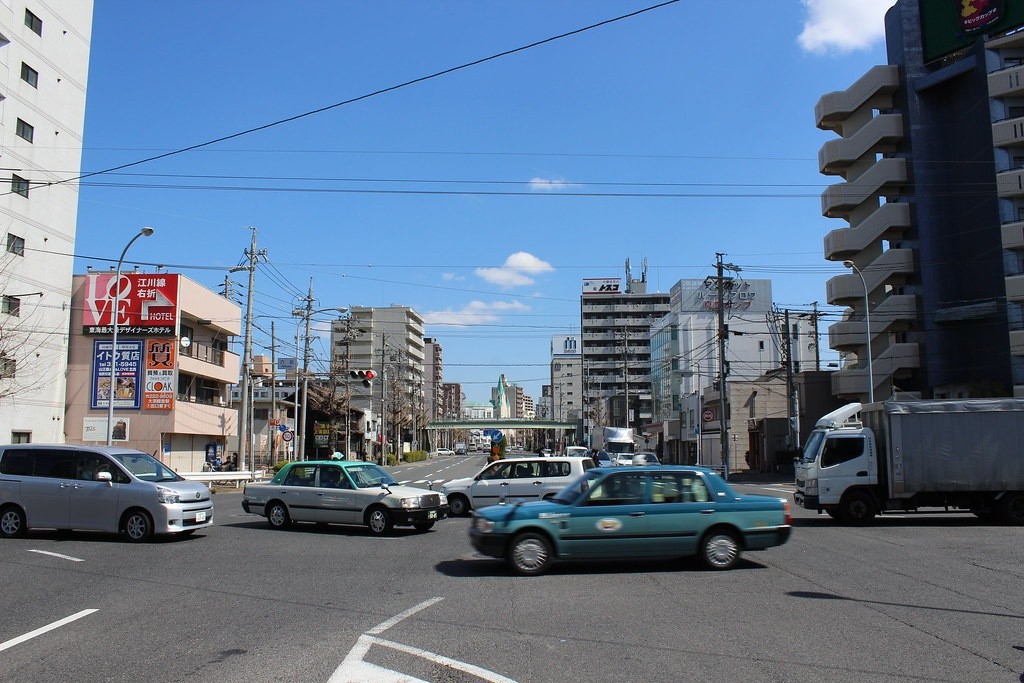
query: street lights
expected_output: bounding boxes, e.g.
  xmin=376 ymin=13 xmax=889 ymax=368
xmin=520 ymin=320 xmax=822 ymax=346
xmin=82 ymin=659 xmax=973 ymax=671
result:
xmin=105 ymin=227 xmax=155 ymax=443
xmin=292 ymin=306 xmax=349 ymax=457
xmin=843 ymin=261 xmax=873 ymax=403
xmin=584 ymin=359 xmax=591 ymax=448
xmin=673 ymin=354 xmax=700 ymax=467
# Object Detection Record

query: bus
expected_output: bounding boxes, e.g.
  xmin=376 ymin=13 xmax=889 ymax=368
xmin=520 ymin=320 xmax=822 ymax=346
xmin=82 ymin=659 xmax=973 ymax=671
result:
xmin=469 ymin=443 xmax=492 ymax=452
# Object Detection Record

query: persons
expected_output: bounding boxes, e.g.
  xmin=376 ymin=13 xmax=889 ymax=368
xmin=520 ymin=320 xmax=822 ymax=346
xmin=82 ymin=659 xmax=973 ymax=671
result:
xmin=81 ymin=457 xmax=97 ymax=480
xmin=210 ymin=452 xmax=238 ymax=472
xmin=361 ymin=449 xmax=368 ymax=463
xmin=330 ymin=470 xmax=341 ymax=488
xmin=588 ymin=448 xmax=595 ymax=458
xmin=592 ymin=450 xmax=602 ymax=467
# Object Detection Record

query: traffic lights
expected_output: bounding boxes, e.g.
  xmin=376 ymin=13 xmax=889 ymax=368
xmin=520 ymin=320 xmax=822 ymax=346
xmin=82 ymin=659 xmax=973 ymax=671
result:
xmin=349 ymin=369 xmax=378 ymax=388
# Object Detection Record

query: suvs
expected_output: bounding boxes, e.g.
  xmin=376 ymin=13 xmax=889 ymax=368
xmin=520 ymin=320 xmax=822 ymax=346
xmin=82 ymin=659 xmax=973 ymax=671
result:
xmin=437 ymin=448 xmax=455 ymax=456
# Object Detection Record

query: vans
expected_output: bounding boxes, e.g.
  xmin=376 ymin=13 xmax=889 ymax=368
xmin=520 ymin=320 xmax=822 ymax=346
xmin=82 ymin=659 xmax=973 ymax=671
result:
xmin=1 ymin=444 xmax=216 ymax=541
xmin=561 ymin=446 xmax=589 ymax=472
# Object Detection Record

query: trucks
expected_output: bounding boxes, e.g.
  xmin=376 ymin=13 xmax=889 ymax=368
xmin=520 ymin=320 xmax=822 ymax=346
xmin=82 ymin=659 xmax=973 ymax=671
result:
xmin=792 ymin=399 xmax=1024 ymax=524
xmin=593 ymin=425 xmax=634 ymax=459
xmin=456 ymin=443 xmax=468 ymax=454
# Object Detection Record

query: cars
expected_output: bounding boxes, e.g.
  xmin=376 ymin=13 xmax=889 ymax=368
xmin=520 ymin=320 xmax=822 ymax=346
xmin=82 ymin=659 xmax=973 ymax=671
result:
xmin=241 ymin=460 xmax=449 ymax=535
xmin=539 ymin=449 xmax=552 ymax=457
xmin=467 ymin=467 xmax=792 ymax=576
xmin=583 ymin=450 xmax=611 ymax=468
xmin=632 ymin=453 xmax=663 ymax=479
xmin=439 ymin=457 xmax=601 ymax=516
xmin=611 ymin=453 xmax=633 ymax=467
